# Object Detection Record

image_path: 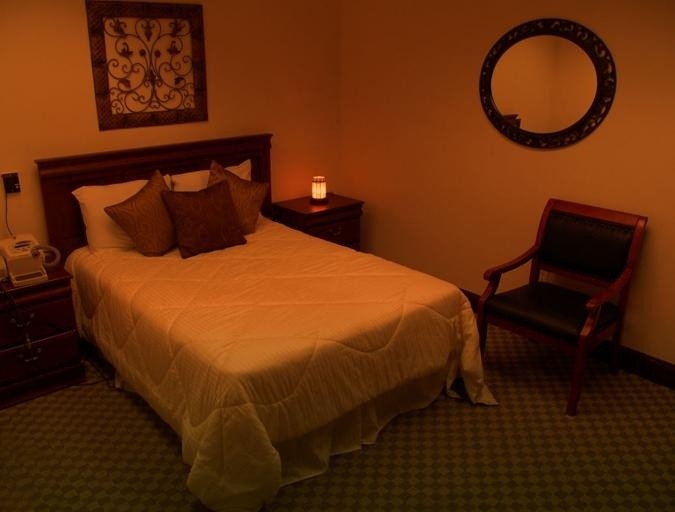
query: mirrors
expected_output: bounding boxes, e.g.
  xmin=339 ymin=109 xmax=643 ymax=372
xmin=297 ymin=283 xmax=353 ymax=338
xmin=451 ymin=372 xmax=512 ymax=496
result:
xmin=479 ymin=16 xmax=615 ymax=152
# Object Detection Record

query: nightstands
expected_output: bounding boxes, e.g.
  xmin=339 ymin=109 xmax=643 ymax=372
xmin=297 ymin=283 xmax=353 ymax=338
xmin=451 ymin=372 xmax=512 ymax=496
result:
xmin=274 ymin=191 xmax=366 ymax=255
xmin=0 ymin=256 xmax=85 ymax=409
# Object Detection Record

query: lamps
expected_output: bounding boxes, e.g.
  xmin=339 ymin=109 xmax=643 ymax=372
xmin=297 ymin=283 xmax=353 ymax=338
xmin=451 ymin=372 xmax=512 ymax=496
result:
xmin=310 ymin=175 xmax=329 ymax=206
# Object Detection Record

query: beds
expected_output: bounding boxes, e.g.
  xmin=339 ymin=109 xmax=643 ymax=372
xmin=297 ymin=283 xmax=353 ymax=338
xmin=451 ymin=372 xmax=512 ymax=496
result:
xmin=30 ymin=129 xmax=480 ymax=510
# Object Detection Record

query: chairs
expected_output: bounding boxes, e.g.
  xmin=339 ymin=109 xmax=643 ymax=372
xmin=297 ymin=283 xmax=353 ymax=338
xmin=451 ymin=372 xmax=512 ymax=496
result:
xmin=472 ymin=194 xmax=649 ymax=418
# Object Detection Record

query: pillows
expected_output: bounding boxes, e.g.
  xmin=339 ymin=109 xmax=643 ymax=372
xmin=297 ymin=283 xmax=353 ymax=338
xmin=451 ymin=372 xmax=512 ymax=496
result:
xmin=61 ymin=159 xmax=268 ymax=260
